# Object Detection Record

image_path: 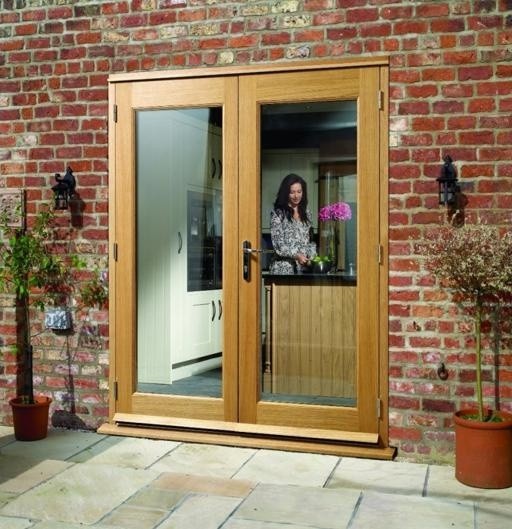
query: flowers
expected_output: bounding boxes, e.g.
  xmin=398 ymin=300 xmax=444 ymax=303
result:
xmin=318 ymin=201 xmax=352 ymax=257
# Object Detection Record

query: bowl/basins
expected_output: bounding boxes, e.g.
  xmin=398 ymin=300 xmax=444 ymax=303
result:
xmin=303 ymin=260 xmax=332 ymax=274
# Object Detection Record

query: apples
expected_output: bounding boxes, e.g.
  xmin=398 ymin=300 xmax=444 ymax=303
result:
xmin=312 ymin=255 xmax=331 ymax=262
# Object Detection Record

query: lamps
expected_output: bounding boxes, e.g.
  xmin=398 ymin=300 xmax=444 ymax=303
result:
xmin=436 ymin=155 xmax=458 ymax=206
xmin=51 ymin=166 xmax=76 ymax=211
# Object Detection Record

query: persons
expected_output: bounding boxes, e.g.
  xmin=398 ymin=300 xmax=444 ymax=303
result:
xmin=267 ymin=174 xmax=318 ymax=276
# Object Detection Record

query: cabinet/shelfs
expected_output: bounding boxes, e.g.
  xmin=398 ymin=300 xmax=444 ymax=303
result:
xmin=137 ymin=109 xmax=187 ymax=386
xmin=187 ymin=111 xmax=222 ymax=191
xmin=187 ymin=288 xmax=225 ymax=378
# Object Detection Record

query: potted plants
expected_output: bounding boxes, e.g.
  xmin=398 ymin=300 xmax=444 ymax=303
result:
xmin=407 ymin=202 xmax=512 ymax=489
xmin=1 ymin=191 xmax=108 ymax=440
